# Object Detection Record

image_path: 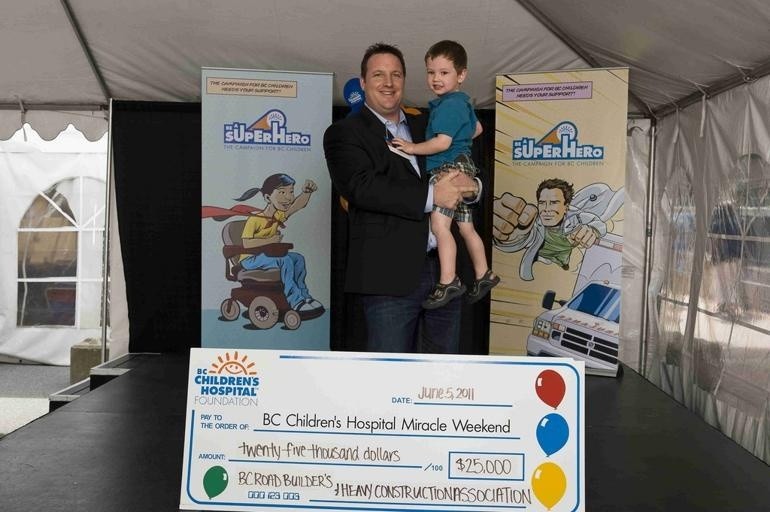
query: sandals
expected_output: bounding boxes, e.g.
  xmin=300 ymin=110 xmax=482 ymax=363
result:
xmin=465 ymin=269 xmax=500 ymax=305
xmin=421 ymin=276 xmax=466 ymax=309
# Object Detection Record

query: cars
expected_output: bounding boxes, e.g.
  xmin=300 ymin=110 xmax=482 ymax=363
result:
xmin=526 ymin=279 xmax=622 ymax=378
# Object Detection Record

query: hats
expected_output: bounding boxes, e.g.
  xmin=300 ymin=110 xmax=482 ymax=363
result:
xmin=344 ymin=78 xmax=365 ymax=113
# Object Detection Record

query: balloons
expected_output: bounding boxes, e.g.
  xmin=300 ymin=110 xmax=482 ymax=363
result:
xmin=535 ymin=413 xmax=569 ymax=456
xmin=203 ymin=465 xmax=228 ymax=499
xmin=536 ymin=369 xmax=565 ymax=410
xmin=531 ymin=463 xmax=566 ymax=510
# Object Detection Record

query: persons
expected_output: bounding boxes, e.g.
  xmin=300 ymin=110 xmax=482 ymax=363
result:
xmin=202 ymin=173 xmax=322 ymax=312
xmin=323 ymin=41 xmax=479 ymax=353
xmin=493 ymin=179 xmax=625 ymax=281
xmin=390 ymin=40 xmax=499 ymax=310
